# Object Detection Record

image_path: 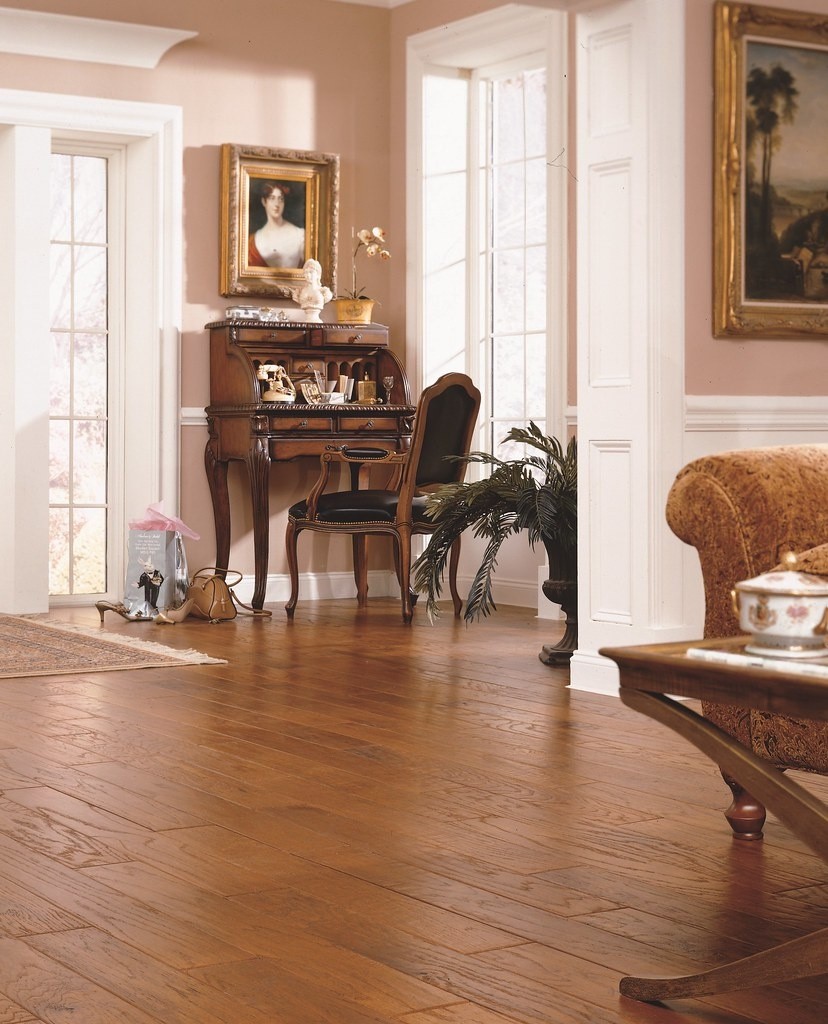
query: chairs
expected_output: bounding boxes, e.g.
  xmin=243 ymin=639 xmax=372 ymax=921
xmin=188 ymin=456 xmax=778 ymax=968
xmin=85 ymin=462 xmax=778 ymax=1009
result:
xmin=284 ymin=372 xmax=482 ymax=626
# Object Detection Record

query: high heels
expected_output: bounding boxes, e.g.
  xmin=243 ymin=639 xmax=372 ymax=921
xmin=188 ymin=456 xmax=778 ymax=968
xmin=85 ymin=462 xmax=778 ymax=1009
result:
xmin=149 ymin=598 xmax=195 ymax=624
xmin=95 ymin=600 xmax=152 ymax=621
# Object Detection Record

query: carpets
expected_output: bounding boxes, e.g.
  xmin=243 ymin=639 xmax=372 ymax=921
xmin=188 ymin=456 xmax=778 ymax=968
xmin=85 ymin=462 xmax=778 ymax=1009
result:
xmin=0 ymin=612 xmax=229 ymax=680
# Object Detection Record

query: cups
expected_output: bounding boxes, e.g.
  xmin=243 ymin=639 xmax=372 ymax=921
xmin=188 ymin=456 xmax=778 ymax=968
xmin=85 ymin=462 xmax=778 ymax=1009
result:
xmin=731 ymin=551 xmax=828 ymax=659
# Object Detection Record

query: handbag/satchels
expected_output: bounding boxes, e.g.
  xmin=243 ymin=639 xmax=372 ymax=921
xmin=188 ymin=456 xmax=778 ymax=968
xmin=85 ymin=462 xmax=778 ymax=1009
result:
xmin=185 ymin=567 xmax=272 ymax=620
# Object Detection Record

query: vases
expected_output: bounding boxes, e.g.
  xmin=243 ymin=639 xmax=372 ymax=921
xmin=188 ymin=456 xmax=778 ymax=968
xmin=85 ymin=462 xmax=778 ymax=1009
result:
xmin=331 ymin=299 xmax=377 ymax=325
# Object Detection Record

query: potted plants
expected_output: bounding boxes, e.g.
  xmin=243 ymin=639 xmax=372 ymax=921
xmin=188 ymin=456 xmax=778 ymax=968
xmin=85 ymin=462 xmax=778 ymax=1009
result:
xmin=412 ymin=419 xmax=578 ymax=666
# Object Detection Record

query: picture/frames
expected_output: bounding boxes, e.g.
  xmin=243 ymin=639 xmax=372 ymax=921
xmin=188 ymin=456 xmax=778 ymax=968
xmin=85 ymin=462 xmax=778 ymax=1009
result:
xmin=710 ymin=1 xmax=828 ymax=343
xmin=219 ymin=143 xmax=341 ymax=300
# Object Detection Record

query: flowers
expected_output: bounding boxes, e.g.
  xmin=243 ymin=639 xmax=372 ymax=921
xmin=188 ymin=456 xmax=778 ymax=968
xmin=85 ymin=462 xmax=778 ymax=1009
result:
xmin=338 ymin=226 xmax=392 ymax=309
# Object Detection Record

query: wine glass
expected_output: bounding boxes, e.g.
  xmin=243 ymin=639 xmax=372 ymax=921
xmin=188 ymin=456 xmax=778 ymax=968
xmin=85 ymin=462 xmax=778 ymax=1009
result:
xmin=382 ymin=376 xmax=394 ymax=406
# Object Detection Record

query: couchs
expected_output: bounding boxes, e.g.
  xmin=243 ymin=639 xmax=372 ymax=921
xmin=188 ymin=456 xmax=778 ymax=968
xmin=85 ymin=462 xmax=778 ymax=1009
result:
xmin=666 ymin=446 xmax=828 ymax=842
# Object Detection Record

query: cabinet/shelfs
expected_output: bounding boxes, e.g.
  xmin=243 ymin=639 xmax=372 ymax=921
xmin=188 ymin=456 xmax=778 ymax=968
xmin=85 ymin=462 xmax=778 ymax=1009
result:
xmin=204 ymin=318 xmax=418 ymax=616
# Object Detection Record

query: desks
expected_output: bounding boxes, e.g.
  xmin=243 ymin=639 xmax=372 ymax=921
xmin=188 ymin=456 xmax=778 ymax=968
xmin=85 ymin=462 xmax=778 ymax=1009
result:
xmin=599 ymin=636 xmax=828 ymax=1001
